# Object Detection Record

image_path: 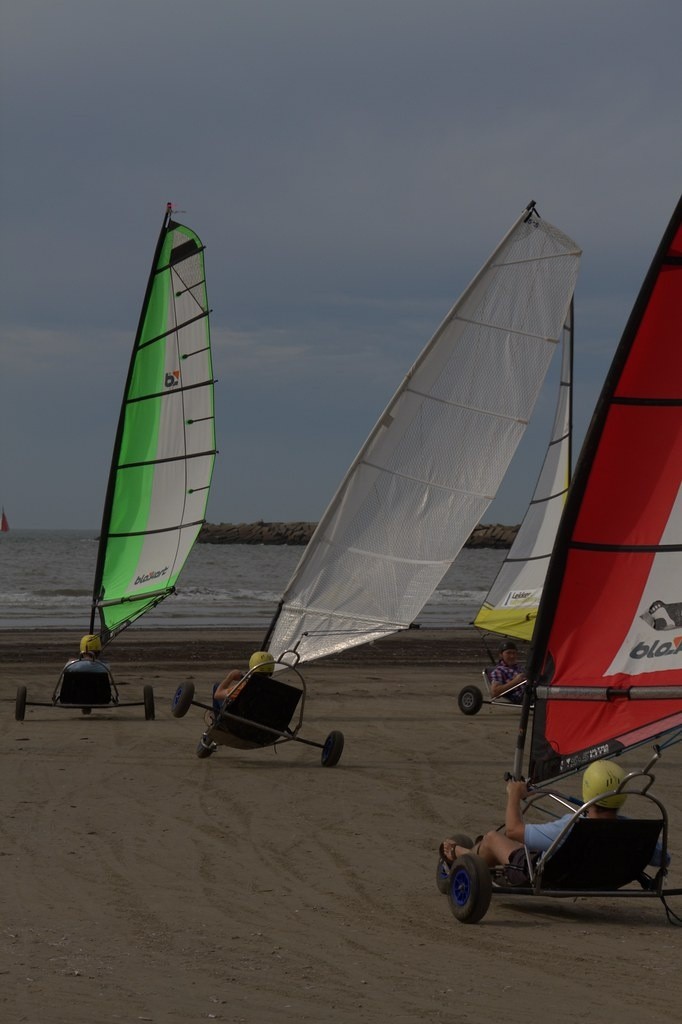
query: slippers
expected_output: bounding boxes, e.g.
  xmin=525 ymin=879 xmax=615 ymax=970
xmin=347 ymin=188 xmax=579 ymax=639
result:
xmin=438 ymin=842 xmax=457 ymax=865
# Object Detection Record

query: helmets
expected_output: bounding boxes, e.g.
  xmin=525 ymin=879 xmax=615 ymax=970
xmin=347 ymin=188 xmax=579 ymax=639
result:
xmin=250 ymin=652 xmax=275 ymax=675
xmin=80 ymin=635 xmax=102 ymax=653
xmin=582 ymin=759 xmax=630 ymax=808
xmin=499 ymin=641 xmax=518 ymax=653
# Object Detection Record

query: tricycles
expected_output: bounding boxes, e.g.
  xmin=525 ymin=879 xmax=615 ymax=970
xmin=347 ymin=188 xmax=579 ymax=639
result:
xmin=435 ymin=753 xmax=682 ymax=925
xmin=457 ymin=668 xmax=534 ymax=716
xmin=172 ymin=651 xmax=344 ymax=769
xmin=14 ymin=645 xmax=159 ymax=722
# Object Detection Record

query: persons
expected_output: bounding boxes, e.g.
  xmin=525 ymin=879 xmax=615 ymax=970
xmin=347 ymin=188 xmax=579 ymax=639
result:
xmin=437 ymin=758 xmax=671 ymax=891
xmin=62 ymin=634 xmax=111 ymax=715
xmin=489 ymin=640 xmax=533 ymax=704
xmin=213 ymin=652 xmax=275 ymax=701
